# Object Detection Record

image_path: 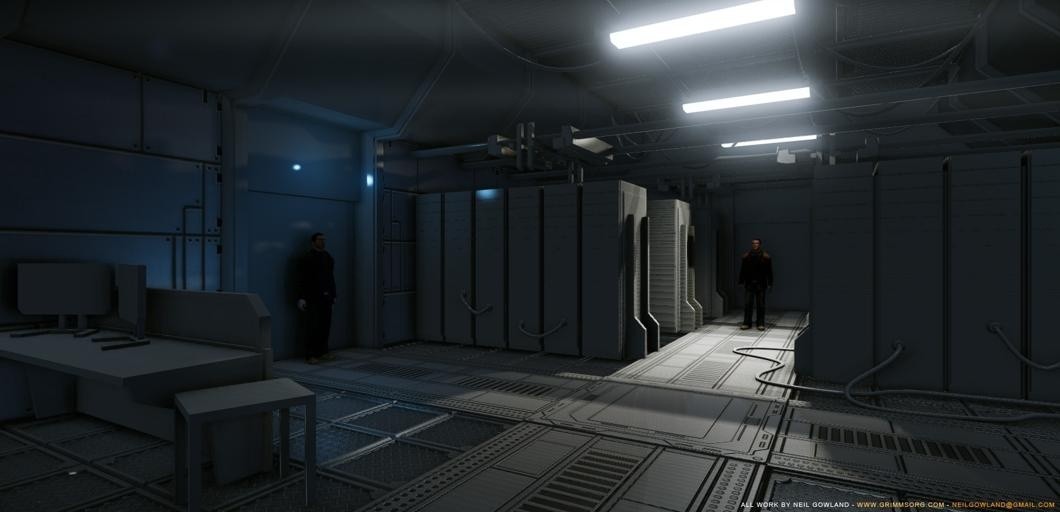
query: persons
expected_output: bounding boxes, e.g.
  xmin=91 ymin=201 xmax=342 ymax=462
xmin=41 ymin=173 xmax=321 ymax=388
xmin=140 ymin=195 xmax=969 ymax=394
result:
xmin=292 ymin=232 xmax=343 ymax=366
xmin=735 ymin=236 xmax=775 ymax=331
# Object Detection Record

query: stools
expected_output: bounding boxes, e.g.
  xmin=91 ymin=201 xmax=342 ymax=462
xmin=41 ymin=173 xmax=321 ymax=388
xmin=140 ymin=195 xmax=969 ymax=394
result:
xmin=174 ymin=376 xmax=316 ymax=512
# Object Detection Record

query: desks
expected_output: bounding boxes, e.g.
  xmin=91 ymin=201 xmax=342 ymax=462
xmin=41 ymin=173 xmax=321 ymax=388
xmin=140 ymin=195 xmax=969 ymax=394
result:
xmin=0 ymin=289 xmax=274 ymax=488
